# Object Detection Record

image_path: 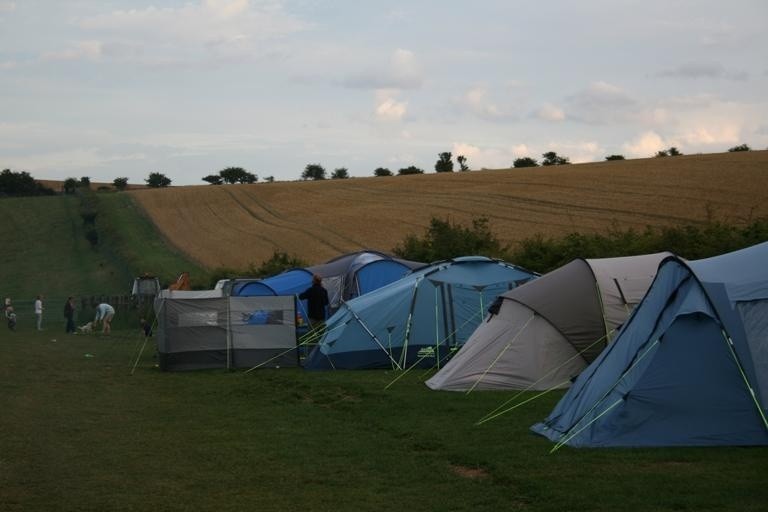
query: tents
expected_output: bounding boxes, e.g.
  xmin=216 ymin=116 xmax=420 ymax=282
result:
xmin=234 ymin=249 xmax=429 ymax=351
xmin=215 ymin=278 xmax=260 ymax=294
xmin=425 ymin=250 xmax=687 ymax=393
xmin=131 ymin=276 xmax=160 ymax=308
xmin=532 ymin=241 xmax=766 ymax=446
xmin=303 ymin=254 xmax=542 ymax=369
xmin=154 ymin=289 xmax=300 ymax=371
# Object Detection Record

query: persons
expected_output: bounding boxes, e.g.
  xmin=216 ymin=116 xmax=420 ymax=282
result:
xmin=5 ymin=305 xmax=16 ymax=329
xmin=92 ymin=302 xmax=115 ymax=336
xmin=3 ymin=296 xmax=11 ymax=310
xmin=34 ymin=296 xmax=44 ymax=331
xmin=299 ymin=273 xmax=329 ymax=332
xmin=64 ymin=296 xmax=78 ymax=334
xmin=169 ymin=270 xmax=190 ymax=291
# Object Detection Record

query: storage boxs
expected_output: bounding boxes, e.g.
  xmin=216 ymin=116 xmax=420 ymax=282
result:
xmin=416 ymin=346 xmax=459 ymax=370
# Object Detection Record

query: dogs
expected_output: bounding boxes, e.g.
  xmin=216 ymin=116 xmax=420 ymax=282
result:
xmin=77 ymin=322 xmax=94 ymax=336
